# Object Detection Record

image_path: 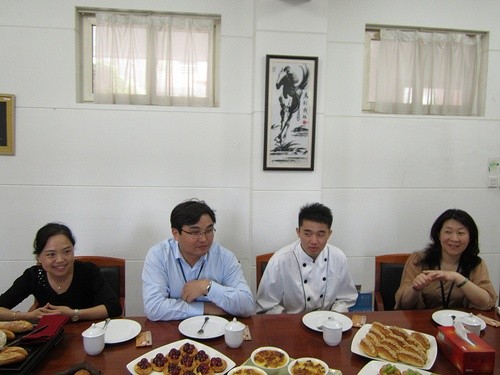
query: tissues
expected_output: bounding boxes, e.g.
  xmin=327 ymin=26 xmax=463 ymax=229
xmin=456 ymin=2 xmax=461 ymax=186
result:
xmin=433 ymin=316 xmax=497 ymax=375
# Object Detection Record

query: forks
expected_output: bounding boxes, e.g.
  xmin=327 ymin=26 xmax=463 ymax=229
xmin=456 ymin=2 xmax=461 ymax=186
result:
xmin=196 ymin=317 xmax=209 ymax=334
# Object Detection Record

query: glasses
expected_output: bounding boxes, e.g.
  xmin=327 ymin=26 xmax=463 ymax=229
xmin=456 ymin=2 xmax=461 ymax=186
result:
xmin=181 ymin=227 xmax=217 ymax=235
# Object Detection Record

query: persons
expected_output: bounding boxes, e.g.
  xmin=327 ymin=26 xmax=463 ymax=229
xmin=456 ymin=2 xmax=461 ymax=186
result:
xmin=394 ymin=209 xmax=498 ymax=311
xmin=0 ymin=224 xmax=123 ymax=324
xmin=142 ymin=200 xmax=255 ymax=322
xmin=255 ymin=203 xmax=359 ymax=315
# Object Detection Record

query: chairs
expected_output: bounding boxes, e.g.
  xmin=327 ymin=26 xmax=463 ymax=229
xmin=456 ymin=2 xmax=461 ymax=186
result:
xmin=28 ymin=256 xmax=126 ymax=317
xmin=256 ymin=253 xmax=274 ymax=290
xmin=374 ymin=253 xmax=411 ymax=311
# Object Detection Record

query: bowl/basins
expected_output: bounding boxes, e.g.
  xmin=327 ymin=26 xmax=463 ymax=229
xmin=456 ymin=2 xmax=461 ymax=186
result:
xmin=288 ymin=357 xmax=329 ymax=375
xmin=251 ymin=346 xmax=289 ymax=375
xmin=227 ymin=365 xmax=268 ymax=375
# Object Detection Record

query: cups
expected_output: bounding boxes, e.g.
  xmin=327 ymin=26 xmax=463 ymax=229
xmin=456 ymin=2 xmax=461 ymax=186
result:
xmin=223 ymin=317 xmax=246 ymax=348
xmin=322 ymin=314 xmax=343 ymax=346
xmin=81 ymin=323 xmax=105 ymax=355
xmin=459 ymin=312 xmax=481 ymax=338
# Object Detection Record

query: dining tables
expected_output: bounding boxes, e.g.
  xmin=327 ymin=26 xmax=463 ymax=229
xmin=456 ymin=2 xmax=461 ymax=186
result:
xmin=30 ymin=310 xmax=500 ymax=375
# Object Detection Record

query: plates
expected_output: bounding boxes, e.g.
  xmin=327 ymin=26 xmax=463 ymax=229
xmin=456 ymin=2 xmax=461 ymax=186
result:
xmin=178 ymin=315 xmax=230 ymax=339
xmin=93 ymin=319 xmax=141 ymax=344
xmin=351 ymin=323 xmax=438 ymax=370
xmin=432 ymin=309 xmax=487 ymax=330
xmin=302 ymin=311 xmax=353 ymax=332
xmin=356 ymin=360 xmax=438 ymax=375
xmin=126 ymin=339 xmax=237 ymax=375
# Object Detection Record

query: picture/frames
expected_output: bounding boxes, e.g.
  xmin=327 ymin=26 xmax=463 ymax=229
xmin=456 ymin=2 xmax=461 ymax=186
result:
xmin=264 ymin=55 xmax=318 ymax=171
xmin=0 ymin=94 xmax=16 ymax=156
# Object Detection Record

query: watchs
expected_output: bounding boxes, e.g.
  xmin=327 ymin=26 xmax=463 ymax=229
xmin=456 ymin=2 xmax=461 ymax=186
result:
xmin=72 ymin=309 xmax=78 ymax=322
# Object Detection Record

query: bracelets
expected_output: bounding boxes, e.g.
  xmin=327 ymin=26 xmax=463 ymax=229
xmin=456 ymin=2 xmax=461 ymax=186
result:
xmin=14 ymin=310 xmax=19 ymax=320
xmin=208 ymin=281 xmax=212 ymax=293
xmin=457 ymin=278 xmax=468 ymax=288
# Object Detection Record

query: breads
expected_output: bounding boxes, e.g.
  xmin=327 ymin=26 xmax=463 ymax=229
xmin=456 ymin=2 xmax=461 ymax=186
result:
xmin=360 ymin=322 xmax=430 ymax=367
xmin=377 ymin=363 xmax=422 ymax=375
xmin=0 ymin=320 xmax=33 ymax=364
xmin=74 ymin=370 xmax=90 ymax=375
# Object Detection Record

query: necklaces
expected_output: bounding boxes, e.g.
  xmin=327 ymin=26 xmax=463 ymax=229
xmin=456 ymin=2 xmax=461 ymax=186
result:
xmin=49 ymin=274 xmax=65 ymax=290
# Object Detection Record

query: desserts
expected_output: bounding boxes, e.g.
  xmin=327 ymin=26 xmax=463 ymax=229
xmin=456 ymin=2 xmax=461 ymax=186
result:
xmin=136 ymin=343 xmax=227 ymax=375
